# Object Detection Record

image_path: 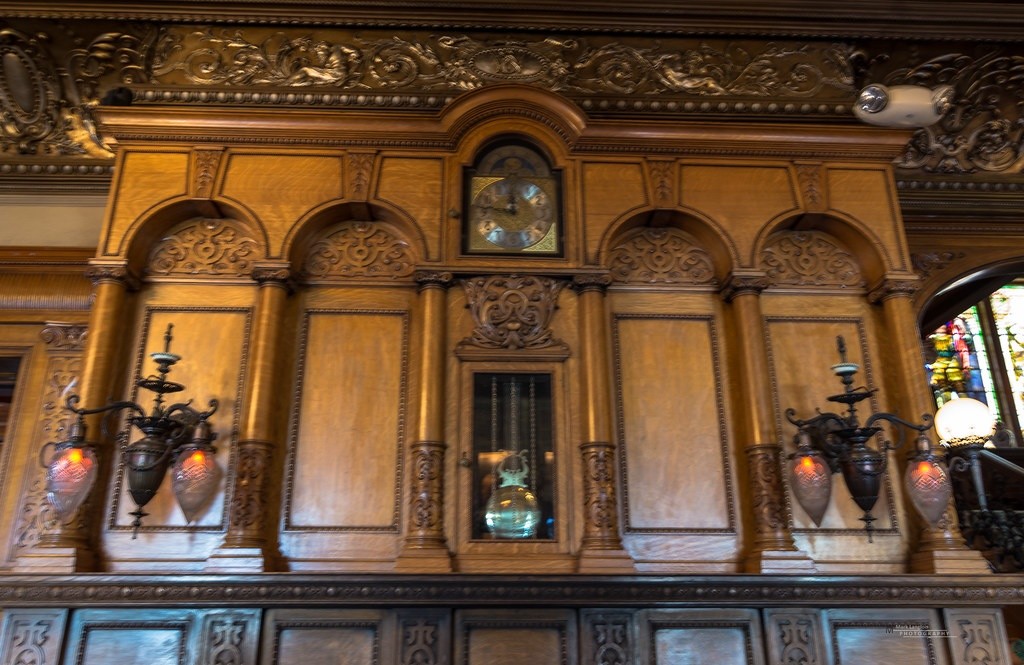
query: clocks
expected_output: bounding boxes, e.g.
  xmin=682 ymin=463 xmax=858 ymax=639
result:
xmin=469 ymin=174 xmax=563 ymax=255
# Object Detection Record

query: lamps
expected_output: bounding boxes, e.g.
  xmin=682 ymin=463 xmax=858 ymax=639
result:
xmin=852 ymin=83 xmax=957 ymax=128
xmin=933 ymin=390 xmax=1000 ymax=515
xmin=39 ymin=324 xmax=221 ymax=539
xmin=785 ymin=335 xmax=952 ymax=544
